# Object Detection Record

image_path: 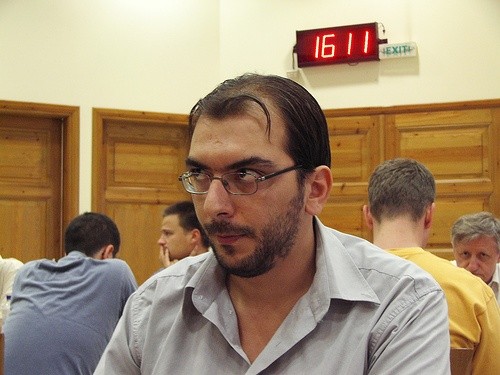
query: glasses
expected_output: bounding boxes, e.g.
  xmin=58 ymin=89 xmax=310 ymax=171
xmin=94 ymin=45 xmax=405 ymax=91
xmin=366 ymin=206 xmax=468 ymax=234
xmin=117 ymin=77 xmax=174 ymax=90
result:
xmin=178 ymin=164 xmax=305 ymax=195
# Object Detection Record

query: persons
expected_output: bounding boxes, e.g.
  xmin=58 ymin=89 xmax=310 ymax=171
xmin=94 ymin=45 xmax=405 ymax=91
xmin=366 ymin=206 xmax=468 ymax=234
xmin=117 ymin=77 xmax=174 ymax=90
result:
xmin=157 ymin=200 xmax=211 ymax=267
xmin=0 ymin=254 xmax=25 ymax=375
xmin=363 ymin=158 xmax=500 ymax=374
xmin=94 ymin=73 xmax=452 ymax=375
xmin=449 ymin=212 xmax=500 ymax=309
xmin=3 ymin=211 xmax=139 ymax=375
